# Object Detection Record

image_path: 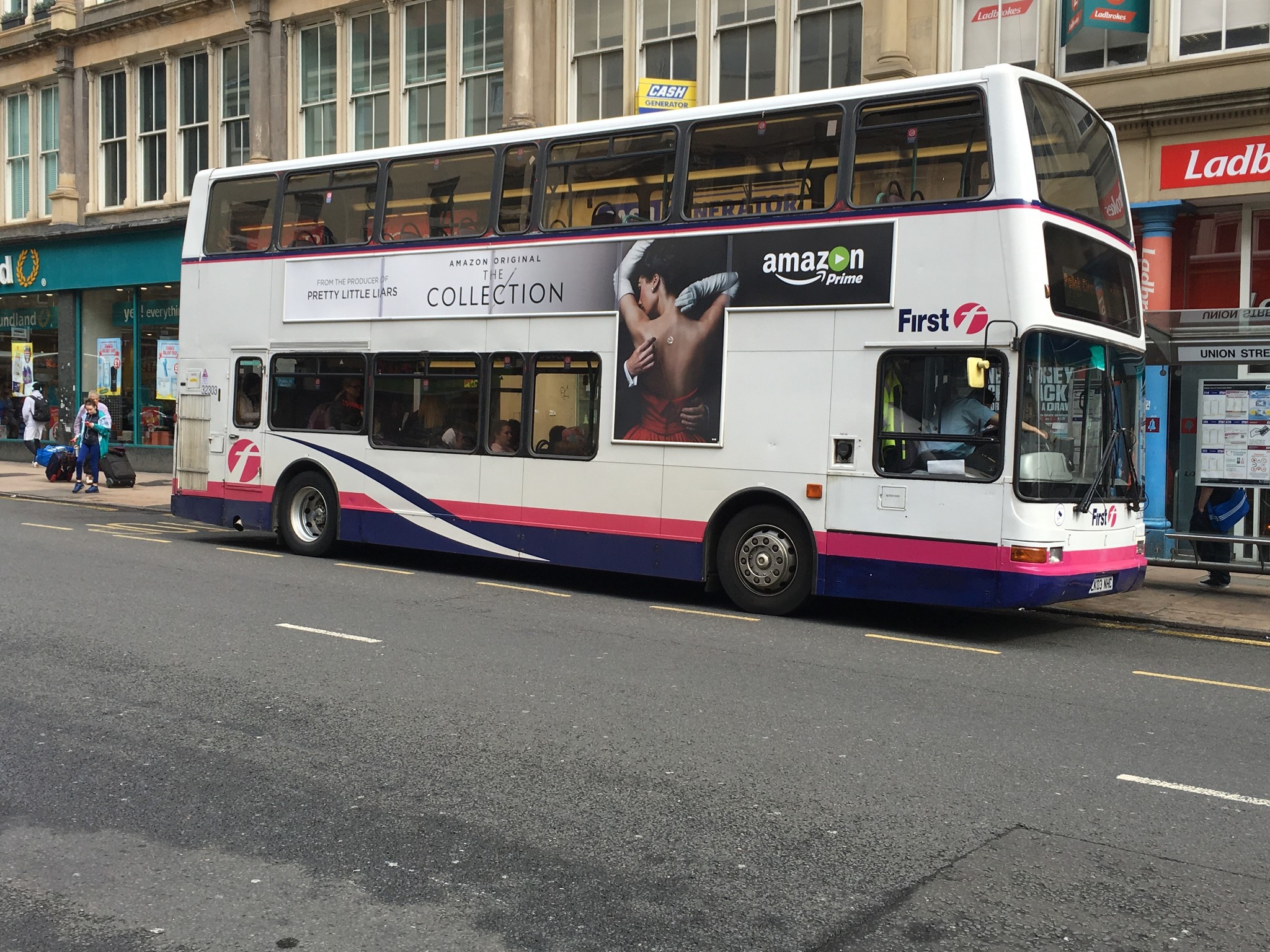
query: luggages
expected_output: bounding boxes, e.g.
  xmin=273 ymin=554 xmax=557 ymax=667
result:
xmin=45 ymin=440 xmax=76 ymax=483
xmin=100 ymin=452 xmax=136 ymax=488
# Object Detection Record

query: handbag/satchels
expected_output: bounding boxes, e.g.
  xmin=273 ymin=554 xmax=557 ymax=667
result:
xmin=1208 ymin=489 xmax=1250 ymax=533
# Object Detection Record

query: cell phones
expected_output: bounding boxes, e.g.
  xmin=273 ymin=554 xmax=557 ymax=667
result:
xmin=85 ymin=421 xmax=90 ymax=425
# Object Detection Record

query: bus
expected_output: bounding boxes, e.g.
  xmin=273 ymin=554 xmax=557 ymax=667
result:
xmin=168 ymin=63 xmax=1145 ymax=619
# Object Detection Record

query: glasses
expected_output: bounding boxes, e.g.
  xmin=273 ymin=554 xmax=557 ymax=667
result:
xmin=348 ymin=384 xmax=363 ymax=389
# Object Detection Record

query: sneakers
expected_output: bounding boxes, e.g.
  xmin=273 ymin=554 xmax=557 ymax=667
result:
xmin=86 ymin=475 xmax=94 ymax=485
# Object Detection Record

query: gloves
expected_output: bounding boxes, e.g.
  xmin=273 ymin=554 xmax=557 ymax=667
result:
xmin=674 ymin=272 xmax=740 ymax=313
xmin=614 ymin=239 xmax=655 ymax=302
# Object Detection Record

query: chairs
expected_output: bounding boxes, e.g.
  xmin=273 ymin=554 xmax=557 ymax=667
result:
xmin=287 ymin=200 xmax=624 ymax=247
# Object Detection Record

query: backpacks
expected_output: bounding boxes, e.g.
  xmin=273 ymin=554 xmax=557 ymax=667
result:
xmin=28 ymin=395 xmax=51 ymax=422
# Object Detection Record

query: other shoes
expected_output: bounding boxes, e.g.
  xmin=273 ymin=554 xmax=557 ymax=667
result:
xmin=32 ymin=461 xmax=39 ymax=467
xmin=1198 ymin=575 xmax=1232 ymax=588
xmin=72 ymin=481 xmax=84 ymax=493
xmin=85 ymin=485 xmax=99 ymax=493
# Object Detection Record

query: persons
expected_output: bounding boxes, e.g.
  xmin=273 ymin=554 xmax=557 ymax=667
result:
xmin=70 ymin=390 xmax=112 ymax=485
xmin=235 ymin=372 xmax=364 ymax=431
xmin=372 ymin=390 xmax=478 ymax=451
xmin=1023 ymin=392 xmax=1051 ymax=440
xmin=22 ymin=382 xmax=47 ymax=467
xmin=1188 ymin=479 xmax=1230 ymax=588
xmin=489 ymin=418 xmax=520 ymax=453
xmin=544 ymin=425 xmax=595 ymax=457
xmin=928 ymin=387 xmax=1046 ymax=474
xmin=612 ymin=238 xmax=739 ymax=443
xmin=0 ymin=373 xmax=58 ymax=439
xmin=73 ymin=399 xmax=111 ymax=493
